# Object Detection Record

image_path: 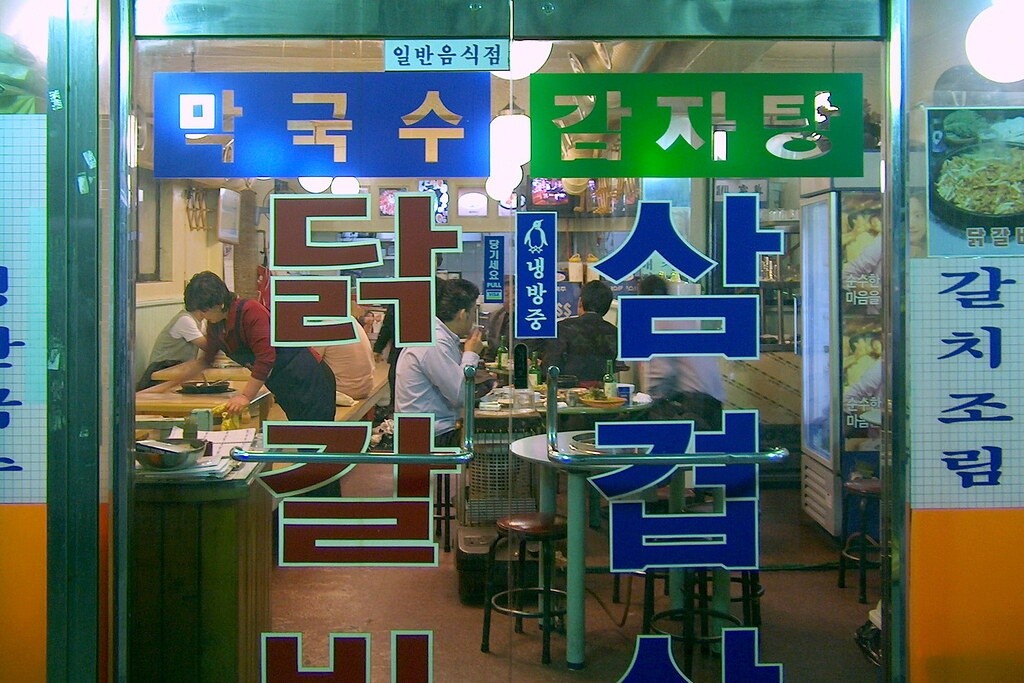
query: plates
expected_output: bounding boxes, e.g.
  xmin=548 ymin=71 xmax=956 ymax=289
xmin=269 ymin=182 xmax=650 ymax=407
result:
xmin=927 ymin=139 xmax=1024 ymax=232
xmin=579 ymin=395 xmax=628 ymax=408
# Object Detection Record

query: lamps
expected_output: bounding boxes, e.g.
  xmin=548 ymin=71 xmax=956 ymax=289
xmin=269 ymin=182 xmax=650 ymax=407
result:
xmin=483 ymin=41 xmax=552 ymax=203
xmin=807 ymin=41 xmax=837 ymax=139
xmin=297 ymin=177 xmax=361 ymax=202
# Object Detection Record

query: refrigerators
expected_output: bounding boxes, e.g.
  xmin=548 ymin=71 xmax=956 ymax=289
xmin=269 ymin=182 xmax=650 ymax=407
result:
xmin=799 ymin=187 xmax=884 ymax=545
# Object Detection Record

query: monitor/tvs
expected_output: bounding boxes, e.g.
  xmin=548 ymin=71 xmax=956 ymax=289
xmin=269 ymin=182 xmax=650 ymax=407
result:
xmin=526 ymin=175 xmax=642 ymax=219
xmin=217 ymin=187 xmax=241 ymax=245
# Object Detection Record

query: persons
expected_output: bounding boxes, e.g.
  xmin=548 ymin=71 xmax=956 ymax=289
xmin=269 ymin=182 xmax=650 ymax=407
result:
xmin=543 ymin=280 xmax=618 ymax=383
xmin=844 ymin=209 xmax=882 ymax=439
xmin=150 ymin=299 xmax=205 ymax=364
xmin=182 ymin=272 xmax=341 ymax=555
xmin=534 ymin=179 xmax=566 ymax=206
xmin=484 ymin=274 xmax=545 ymax=362
xmin=308 ymin=315 xmax=375 ymax=400
xmin=910 ymin=189 xmax=928 ymax=259
xmin=373 ymin=251 xmax=443 ymax=413
xmin=395 ymin=278 xmax=484 ymax=507
xmin=637 ymin=275 xmax=724 ymax=432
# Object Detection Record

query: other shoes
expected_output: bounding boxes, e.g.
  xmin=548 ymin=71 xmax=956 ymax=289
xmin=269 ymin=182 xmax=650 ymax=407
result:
xmin=452 ymin=493 xmax=470 ymax=525
xmin=852 ymin=619 xmax=883 ymax=666
xmin=381 ymin=405 xmax=395 ymax=413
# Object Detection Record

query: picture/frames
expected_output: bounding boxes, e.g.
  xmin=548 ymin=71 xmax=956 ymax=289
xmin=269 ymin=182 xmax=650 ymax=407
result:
xmin=378 ymin=184 xmax=410 ymax=218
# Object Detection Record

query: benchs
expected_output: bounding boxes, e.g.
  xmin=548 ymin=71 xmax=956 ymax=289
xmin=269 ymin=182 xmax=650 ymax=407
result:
xmin=337 ymin=362 xmax=392 ymax=422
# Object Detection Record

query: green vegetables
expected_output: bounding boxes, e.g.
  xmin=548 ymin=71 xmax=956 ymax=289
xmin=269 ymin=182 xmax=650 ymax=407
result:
xmin=582 ymin=388 xmax=609 ymax=401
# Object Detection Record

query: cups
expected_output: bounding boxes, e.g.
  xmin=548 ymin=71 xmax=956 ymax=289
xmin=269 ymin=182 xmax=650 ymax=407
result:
xmin=616 ymin=383 xmax=636 ymax=404
xmin=203 ymin=442 xmax=212 ymax=456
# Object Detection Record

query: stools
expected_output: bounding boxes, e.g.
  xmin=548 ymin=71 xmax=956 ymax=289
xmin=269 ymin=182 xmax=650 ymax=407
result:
xmin=839 ymin=480 xmax=883 ymax=602
xmin=430 ymin=473 xmax=464 ymax=553
xmin=630 ymin=486 xmax=695 ymax=595
xmin=481 ymin=514 xmax=572 ymax=666
xmin=648 ymin=565 xmax=743 ymax=681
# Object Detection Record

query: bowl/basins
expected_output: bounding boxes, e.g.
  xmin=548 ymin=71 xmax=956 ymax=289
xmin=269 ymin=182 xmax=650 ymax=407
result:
xmin=135 ymin=437 xmax=206 ymax=471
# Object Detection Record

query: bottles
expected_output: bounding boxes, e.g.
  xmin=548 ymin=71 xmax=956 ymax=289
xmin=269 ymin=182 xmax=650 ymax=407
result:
xmin=602 ymin=360 xmax=618 ymax=398
xmin=529 ymin=351 xmax=542 ymax=386
xmin=496 ymin=335 xmax=509 ymax=369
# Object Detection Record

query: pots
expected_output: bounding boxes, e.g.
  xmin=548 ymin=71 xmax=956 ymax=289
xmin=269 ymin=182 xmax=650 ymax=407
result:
xmin=179 ymin=382 xmax=230 ymax=393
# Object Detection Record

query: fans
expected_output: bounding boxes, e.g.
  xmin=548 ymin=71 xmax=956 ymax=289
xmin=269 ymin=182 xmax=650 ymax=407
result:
xmin=254 ymin=186 xmax=297 ymax=225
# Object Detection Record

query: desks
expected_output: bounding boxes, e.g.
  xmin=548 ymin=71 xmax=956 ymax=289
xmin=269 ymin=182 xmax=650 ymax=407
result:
xmin=469 ymin=389 xmax=654 ymax=532
xmin=132 ymin=382 xmax=272 ymax=432
xmin=862 ymin=409 xmax=894 ymax=430
xmin=510 ymin=433 xmax=736 ymax=677
xmin=150 ymin=356 xmax=258 ymax=382
